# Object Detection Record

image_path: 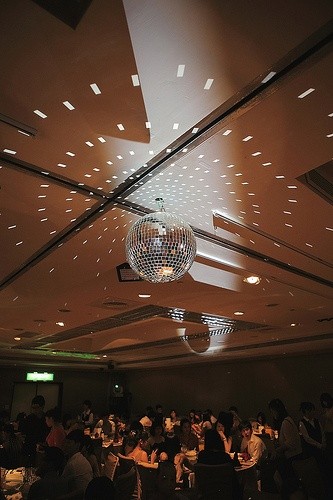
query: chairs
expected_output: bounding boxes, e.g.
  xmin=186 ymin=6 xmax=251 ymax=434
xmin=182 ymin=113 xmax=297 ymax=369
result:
xmin=102 ymin=451 xmax=240 ymax=500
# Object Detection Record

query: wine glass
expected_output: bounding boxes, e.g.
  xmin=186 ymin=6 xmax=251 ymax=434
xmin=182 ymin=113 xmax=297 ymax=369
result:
xmin=181 ymin=447 xmax=187 ymax=455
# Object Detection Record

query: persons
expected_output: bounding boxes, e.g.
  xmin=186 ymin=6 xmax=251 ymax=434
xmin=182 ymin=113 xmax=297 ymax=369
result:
xmin=0 ymin=396 xmax=333 ymax=500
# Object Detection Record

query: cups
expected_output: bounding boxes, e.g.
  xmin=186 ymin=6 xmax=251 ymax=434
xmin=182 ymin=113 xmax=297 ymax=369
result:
xmin=166 ymin=418 xmax=171 ymax=428
xmin=84 ymin=431 xmax=89 ymax=435
xmin=259 ymin=426 xmax=264 ymax=434
xmin=94 ymin=427 xmax=101 ymax=439
xmin=22 ymin=468 xmax=33 ymax=484
xmin=1 ymin=468 xmax=6 ymax=489
xmin=198 ymin=440 xmax=204 ymax=451
xmin=265 ymin=428 xmax=272 ymax=438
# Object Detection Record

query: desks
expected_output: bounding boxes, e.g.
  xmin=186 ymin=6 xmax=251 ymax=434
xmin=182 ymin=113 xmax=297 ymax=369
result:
xmin=0 ymin=467 xmax=39 ymax=500
xmin=235 ymin=460 xmax=258 ymax=500
